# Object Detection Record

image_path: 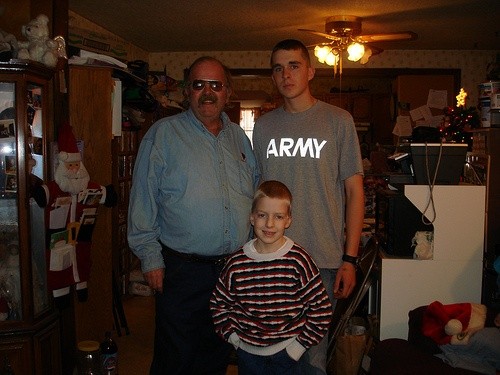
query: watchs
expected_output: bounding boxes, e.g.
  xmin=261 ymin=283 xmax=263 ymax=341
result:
xmin=342 ymin=254 xmax=363 ymax=268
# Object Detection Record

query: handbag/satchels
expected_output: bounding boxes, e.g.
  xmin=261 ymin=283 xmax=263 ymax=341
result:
xmin=335 ymin=334 xmax=370 ymax=374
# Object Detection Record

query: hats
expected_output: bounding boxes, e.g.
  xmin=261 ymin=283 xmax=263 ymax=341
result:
xmin=423 ymin=301 xmax=486 ymax=347
xmin=434 ymin=327 xmax=500 ymax=375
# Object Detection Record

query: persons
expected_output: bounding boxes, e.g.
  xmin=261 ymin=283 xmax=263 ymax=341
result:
xmin=127 ymin=54 xmax=261 ymax=375
xmin=210 ymin=180 xmax=334 ymax=375
xmin=252 ymin=38 xmax=366 ymax=375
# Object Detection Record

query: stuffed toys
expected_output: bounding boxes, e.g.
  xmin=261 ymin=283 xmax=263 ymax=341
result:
xmin=12 ymin=14 xmax=58 ymax=67
xmin=30 ymin=124 xmax=119 ymax=312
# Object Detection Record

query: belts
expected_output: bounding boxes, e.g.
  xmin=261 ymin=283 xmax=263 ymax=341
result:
xmin=157 ymin=240 xmax=227 ymax=267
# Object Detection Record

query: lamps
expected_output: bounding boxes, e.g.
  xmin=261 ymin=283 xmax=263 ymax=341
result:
xmin=314 ymin=15 xmax=372 ymax=67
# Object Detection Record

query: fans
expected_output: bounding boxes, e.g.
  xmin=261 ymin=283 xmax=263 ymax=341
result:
xmin=297 ymin=15 xmax=411 ymax=50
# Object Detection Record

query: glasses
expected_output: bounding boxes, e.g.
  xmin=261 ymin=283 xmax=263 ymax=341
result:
xmin=187 ymin=80 xmax=228 ymax=91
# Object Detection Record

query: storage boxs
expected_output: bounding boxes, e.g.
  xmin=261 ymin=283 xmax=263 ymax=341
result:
xmin=479 ymin=82 xmax=500 ymax=127
xmin=402 ymin=141 xmax=468 ymax=185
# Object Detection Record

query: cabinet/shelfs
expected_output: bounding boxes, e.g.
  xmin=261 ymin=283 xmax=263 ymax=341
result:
xmin=463 ymin=127 xmax=500 ymax=268
xmin=67 ymin=64 xmax=183 ymax=343
xmin=368 ymin=182 xmax=485 ymax=342
xmin=0 ymin=58 xmax=68 ymax=375
